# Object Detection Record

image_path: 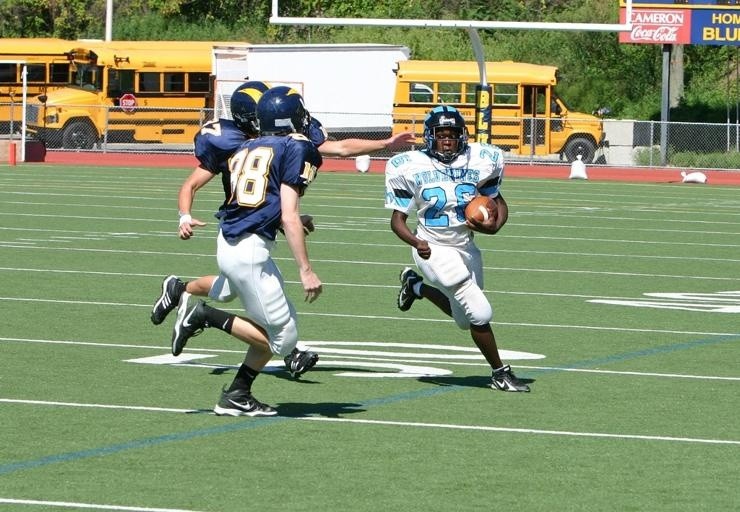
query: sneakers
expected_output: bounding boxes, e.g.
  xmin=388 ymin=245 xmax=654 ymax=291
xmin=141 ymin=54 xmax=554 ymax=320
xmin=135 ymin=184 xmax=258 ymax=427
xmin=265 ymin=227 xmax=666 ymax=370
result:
xmin=152 ymin=276 xmax=210 ymax=356
xmin=284 ymin=347 xmax=319 ymax=378
xmin=491 ymin=365 xmax=530 ymax=392
xmin=397 ymin=266 xmax=423 ymax=311
xmin=214 ymin=384 xmax=278 ymax=417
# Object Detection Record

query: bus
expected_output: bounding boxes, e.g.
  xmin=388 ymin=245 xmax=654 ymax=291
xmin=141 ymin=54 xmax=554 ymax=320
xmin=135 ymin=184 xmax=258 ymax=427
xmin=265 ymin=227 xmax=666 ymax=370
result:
xmin=23 ymin=40 xmax=251 ymax=157
xmin=392 ymin=61 xmax=609 ymax=166
xmin=0 ymin=38 xmax=96 ymax=127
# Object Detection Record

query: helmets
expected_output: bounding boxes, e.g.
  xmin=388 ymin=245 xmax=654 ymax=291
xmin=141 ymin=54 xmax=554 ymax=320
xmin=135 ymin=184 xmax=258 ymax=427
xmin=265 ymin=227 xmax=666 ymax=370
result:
xmin=423 ymin=106 xmax=468 ymax=163
xmin=230 ymin=81 xmax=311 ymax=139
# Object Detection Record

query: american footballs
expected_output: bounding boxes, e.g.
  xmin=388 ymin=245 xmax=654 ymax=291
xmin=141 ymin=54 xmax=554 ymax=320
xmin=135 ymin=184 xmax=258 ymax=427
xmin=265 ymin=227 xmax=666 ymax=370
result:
xmin=464 ymin=195 xmax=498 ymax=223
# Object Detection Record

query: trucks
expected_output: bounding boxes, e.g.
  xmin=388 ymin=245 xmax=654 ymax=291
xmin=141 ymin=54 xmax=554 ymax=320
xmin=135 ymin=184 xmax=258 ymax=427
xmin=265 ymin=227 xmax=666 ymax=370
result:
xmin=213 ymin=43 xmax=444 ymax=138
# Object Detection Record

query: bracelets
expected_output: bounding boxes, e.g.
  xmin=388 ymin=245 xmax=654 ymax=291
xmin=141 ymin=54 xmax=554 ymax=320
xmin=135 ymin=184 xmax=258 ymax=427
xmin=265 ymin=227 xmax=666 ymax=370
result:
xmin=177 ymin=214 xmax=193 ymax=227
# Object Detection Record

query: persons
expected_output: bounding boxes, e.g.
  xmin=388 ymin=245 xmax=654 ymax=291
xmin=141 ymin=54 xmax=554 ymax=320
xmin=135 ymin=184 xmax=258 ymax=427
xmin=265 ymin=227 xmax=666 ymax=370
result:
xmin=150 ymin=81 xmax=416 ymax=379
xmin=384 ymin=105 xmax=531 ymax=392
xmin=171 ymin=87 xmax=322 ymax=417
